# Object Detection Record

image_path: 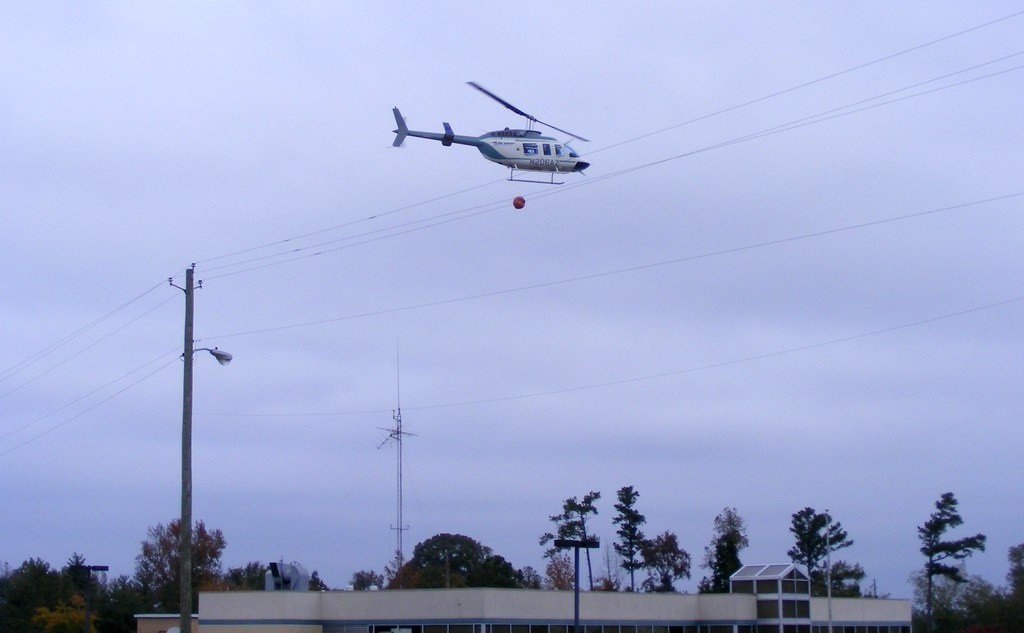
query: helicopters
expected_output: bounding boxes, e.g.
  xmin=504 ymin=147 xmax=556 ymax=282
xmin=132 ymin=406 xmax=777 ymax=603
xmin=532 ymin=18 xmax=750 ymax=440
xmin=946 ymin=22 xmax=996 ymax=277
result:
xmin=393 ymin=82 xmax=591 ymax=186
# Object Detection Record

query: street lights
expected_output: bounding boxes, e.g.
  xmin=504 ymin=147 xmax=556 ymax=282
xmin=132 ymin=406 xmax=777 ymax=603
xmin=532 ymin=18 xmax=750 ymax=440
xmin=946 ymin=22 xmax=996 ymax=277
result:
xmin=179 ymin=346 xmax=233 ymax=633
xmin=554 ymin=539 xmax=600 ymax=633
xmin=70 ymin=565 xmax=108 ymax=633
